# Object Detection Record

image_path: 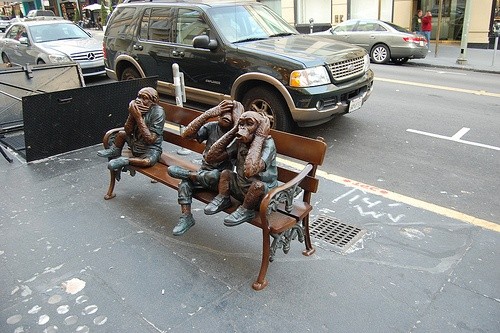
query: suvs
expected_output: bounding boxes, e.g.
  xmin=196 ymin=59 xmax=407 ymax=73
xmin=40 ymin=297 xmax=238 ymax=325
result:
xmin=102 ymin=1 xmax=375 ymax=133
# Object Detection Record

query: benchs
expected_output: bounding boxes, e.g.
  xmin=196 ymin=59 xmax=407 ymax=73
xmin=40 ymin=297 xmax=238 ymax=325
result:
xmin=103 ymin=99 xmax=328 ymax=290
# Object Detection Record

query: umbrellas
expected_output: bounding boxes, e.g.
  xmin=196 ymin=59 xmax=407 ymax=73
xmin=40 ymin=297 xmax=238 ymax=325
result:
xmin=83 ymin=3 xmax=110 ymax=11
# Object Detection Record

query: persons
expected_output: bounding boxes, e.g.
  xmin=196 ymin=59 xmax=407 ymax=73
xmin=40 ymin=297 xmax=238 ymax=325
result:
xmin=413 ymin=9 xmax=423 ymax=34
xmin=422 ymin=10 xmax=433 ymax=52
xmin=16 ymin=28 xmax=27 ymax=41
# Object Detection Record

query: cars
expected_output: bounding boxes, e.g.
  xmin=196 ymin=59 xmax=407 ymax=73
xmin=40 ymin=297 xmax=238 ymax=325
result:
xmin=0 ymin=10 xmax=64 ymax=33
xmin=0 ymin=20 xmax=107 ymax=78
xmin=308 ymin=19 xmax=429 ymax=66
